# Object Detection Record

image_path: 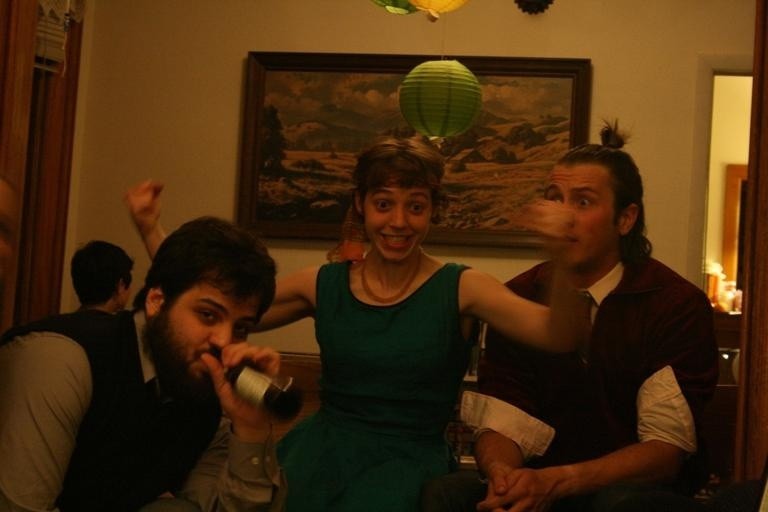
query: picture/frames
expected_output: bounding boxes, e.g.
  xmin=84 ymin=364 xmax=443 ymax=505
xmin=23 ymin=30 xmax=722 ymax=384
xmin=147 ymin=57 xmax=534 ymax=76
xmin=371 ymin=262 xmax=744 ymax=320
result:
xmin=235 ymin=50 xmax=591 ymax=249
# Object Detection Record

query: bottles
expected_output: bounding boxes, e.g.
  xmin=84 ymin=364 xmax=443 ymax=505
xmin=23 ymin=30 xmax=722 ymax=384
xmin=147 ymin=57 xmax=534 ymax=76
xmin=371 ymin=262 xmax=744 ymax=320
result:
xmin=207 ymin=346 xmax=303 ymax=421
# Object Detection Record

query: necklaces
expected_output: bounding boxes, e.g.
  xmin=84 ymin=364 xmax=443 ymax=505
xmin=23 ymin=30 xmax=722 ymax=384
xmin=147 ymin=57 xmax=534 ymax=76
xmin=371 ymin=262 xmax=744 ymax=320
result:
xmin=360 ymin=248 xmax=422 ymax=304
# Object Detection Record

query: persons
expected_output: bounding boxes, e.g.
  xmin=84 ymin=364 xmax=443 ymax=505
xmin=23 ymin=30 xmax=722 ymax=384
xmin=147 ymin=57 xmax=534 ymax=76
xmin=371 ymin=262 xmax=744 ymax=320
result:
xmin=0 ymin=216 xmax=289 ymax=512
xmin=123 ymin=136 xmax=590 ymax=512
xmin=70 ymin=240 xmax=135 ymax=316
xmin=418 ymin=118 xmax=719 ymax=512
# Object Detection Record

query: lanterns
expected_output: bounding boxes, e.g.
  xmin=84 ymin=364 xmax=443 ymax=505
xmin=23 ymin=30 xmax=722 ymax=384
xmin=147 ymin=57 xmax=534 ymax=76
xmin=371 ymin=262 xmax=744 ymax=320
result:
xmin=408 ymin=0 xmax=469 ymax=22
xmin=398 ymin=60 xmax=482 ymax=149
xmin=372 ymin=0 xmax=417 ymax=15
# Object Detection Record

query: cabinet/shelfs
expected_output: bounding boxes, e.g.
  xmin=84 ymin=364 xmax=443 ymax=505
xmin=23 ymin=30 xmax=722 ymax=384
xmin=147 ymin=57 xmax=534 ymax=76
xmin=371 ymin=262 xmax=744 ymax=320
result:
xmin=697 ymin=311 xmax=744 ymax=477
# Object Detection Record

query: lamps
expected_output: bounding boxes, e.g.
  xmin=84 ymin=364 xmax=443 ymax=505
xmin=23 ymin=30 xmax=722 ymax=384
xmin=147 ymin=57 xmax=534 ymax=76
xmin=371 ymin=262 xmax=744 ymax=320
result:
xmin=372 ymin=0 xmax=481 ymax=147
